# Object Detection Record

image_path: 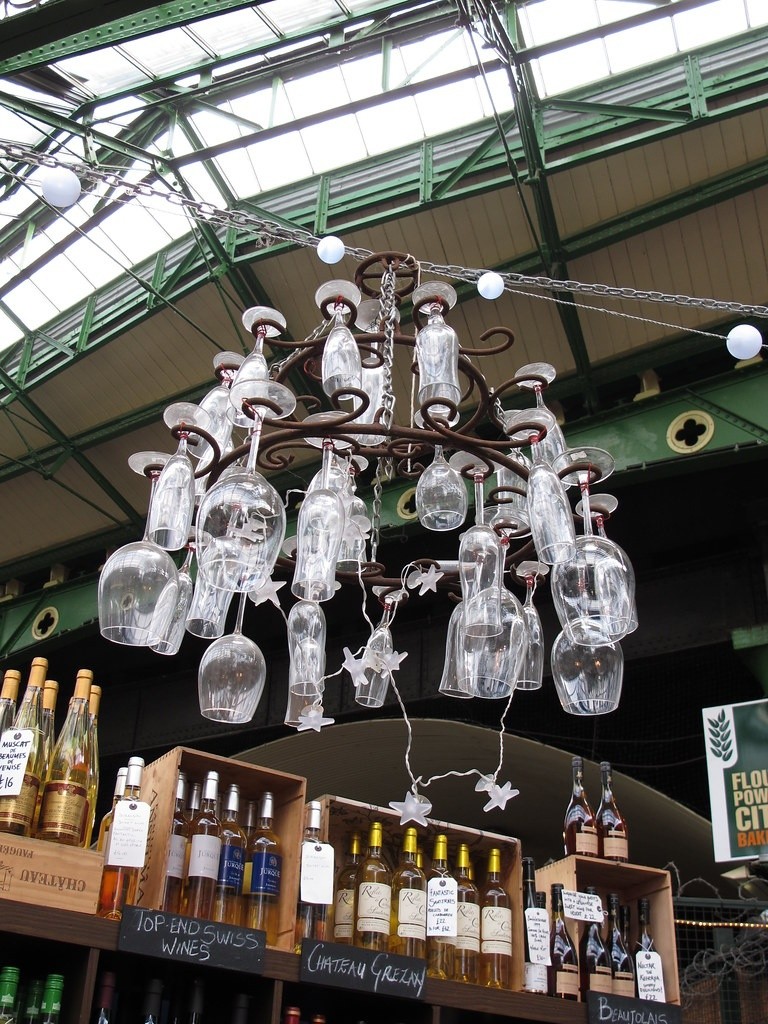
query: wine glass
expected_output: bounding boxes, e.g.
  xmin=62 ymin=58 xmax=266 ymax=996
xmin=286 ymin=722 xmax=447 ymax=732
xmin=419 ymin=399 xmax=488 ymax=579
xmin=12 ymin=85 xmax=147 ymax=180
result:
xmin=96 ymin=279 xmax=641 ymax=724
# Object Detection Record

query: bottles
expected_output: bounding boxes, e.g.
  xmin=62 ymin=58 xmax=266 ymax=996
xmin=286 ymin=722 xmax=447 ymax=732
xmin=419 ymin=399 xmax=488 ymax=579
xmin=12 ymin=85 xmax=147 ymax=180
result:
xmin=0 ymin=655 xmax=669 ymax=1023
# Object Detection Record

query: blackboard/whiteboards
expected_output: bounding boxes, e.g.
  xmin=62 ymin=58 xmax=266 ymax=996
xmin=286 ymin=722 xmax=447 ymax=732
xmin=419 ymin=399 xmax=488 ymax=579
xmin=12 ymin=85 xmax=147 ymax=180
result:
xmin=587 ymin=991 xmax=681 ymax=1024
xmin=116 ymin=904 xmax=266 ymax=975
xmin=299 ymin=937 xmax=427 ymax=1001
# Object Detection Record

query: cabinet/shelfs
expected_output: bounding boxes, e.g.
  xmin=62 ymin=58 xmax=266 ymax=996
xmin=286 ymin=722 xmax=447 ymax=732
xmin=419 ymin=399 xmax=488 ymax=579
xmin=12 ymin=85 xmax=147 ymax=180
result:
xmin=0 ymin=898 xmax=587 ymax=1024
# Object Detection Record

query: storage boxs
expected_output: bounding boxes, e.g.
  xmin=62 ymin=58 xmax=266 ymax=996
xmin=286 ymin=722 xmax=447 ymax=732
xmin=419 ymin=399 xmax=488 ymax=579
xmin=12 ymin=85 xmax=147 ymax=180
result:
xmin=0 ymin=831 xmax=105 ymax=915
xmin=535 ymin=853 xmax=682 ymax=1006
xmin=305 ymin=792 xmax=525 ymax=993
xmin=126 ymin=743 xmax=309 ymax=952
